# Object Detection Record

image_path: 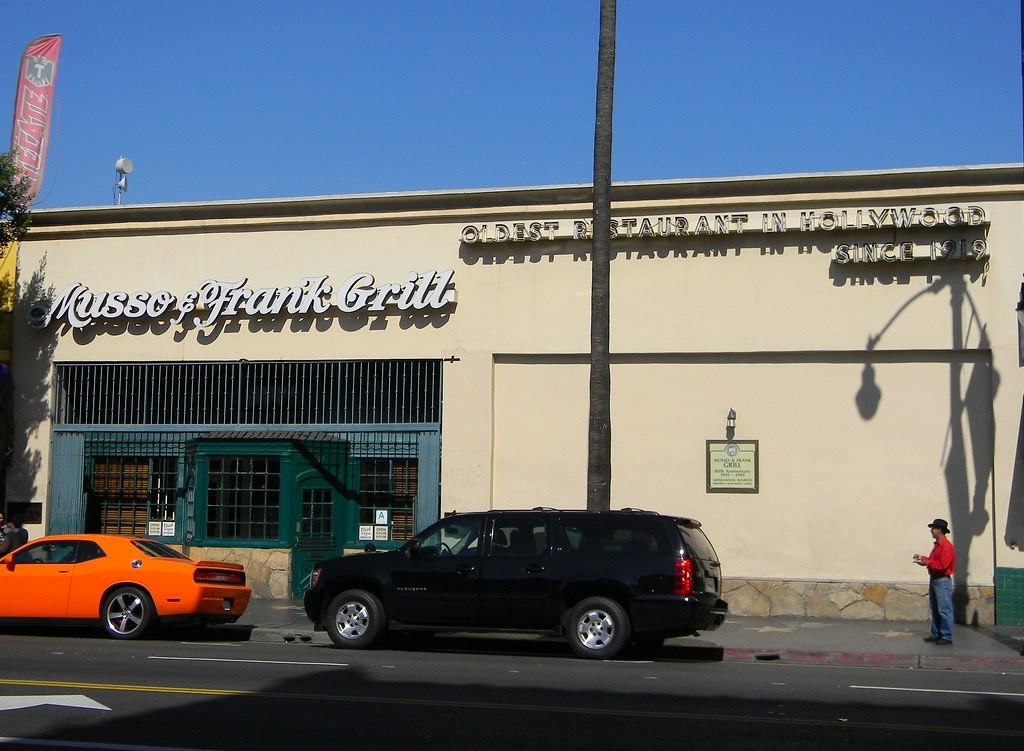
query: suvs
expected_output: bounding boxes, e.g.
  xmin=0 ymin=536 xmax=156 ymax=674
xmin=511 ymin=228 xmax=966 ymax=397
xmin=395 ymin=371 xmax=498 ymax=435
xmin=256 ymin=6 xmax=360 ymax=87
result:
xmin=302 ymin=505 xmax=731 ymax=660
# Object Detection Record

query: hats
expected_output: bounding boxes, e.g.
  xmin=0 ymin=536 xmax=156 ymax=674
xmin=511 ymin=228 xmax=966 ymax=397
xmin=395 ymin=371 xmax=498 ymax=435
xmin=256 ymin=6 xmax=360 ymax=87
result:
xmin=928 ymin=519 xmax=950 ymax=534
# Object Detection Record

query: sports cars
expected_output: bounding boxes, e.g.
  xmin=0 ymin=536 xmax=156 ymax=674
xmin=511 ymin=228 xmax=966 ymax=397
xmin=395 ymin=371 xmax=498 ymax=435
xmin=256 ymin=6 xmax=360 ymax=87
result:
xmin=0 ymin=533 xmax=253 ymax=639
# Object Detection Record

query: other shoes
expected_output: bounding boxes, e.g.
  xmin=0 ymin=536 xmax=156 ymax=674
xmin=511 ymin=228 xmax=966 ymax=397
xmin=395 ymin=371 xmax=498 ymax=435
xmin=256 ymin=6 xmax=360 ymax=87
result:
xmin=924 ymin=635 xmax=940 ymax=642
xmin=935 ymin=638 xmax=952 ymax=645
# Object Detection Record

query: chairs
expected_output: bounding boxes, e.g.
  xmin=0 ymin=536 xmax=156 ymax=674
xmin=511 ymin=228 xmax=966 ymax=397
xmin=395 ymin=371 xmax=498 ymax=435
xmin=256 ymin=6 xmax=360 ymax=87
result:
xmin=493 ymin=529 xmax=508 ymax=552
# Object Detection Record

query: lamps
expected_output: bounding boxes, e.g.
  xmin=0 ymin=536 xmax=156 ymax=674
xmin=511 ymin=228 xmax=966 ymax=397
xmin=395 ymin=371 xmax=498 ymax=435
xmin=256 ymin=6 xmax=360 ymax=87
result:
xmin=726 ymin=407 xmax=737 ymax=431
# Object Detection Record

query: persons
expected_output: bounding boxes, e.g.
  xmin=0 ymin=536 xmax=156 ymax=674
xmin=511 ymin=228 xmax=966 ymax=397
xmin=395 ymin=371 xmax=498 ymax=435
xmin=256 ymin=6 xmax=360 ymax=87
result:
xmin=913 ymin=519 xmax=955 ymax=645
xmin=0 ymin=514 xmax=29 ymax=558
